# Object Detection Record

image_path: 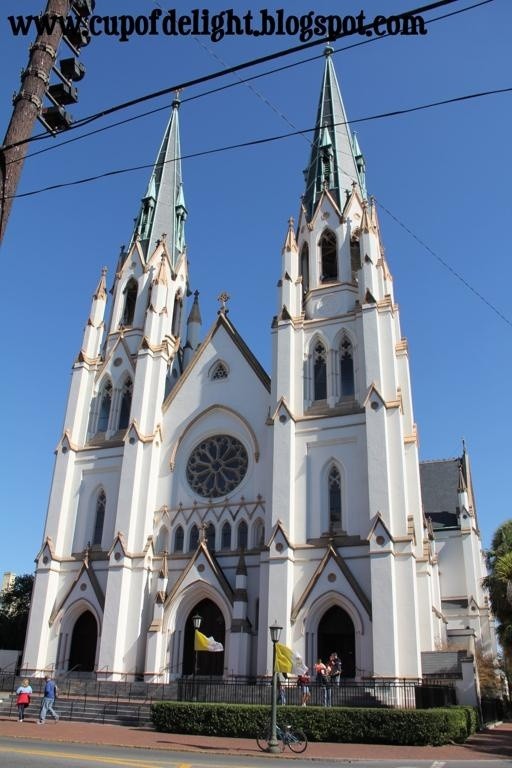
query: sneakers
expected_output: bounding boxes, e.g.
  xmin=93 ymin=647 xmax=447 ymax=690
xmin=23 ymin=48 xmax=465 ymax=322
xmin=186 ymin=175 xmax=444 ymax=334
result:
xmin=39 ymin=716 xmax=59 ymax=724
xmin=301 ymin=703 xmax=306 ymax=706
xmin=17 ymin=719 xmax=24 ymax=722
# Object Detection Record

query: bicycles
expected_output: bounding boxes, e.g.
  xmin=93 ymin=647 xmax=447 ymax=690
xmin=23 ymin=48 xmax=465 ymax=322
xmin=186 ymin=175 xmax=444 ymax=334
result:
xmin=256 ymin=712 xmax=308 ymax=753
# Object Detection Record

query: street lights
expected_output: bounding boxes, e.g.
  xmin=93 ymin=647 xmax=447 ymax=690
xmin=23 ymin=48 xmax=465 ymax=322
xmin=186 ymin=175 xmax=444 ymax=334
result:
xmin=266 ymin=620 xmax=283 ymax=753
xmin=192 ymin=613 xmax=202 ymax=702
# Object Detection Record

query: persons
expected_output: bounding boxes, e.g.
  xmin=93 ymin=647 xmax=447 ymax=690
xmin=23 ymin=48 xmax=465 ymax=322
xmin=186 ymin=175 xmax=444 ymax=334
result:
xmin=36 ymin=673 xmax=60 ymax=724
xmin=276 ymin=670 xmax=290 ymax=704
xmin=314 ymin=651 xmax=342 ymax=708
xmin=15 ymin=678 xmax=32 ymax=722
xmin=297 ymin=665 xmax=311 ymax=706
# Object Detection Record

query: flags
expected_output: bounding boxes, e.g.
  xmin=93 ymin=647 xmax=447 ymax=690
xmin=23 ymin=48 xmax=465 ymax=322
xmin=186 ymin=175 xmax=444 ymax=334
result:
xmin=193 ymin=629 xmax=224 ymax=652
xmin=274 ymin=641 xmax=309 ymax=676
